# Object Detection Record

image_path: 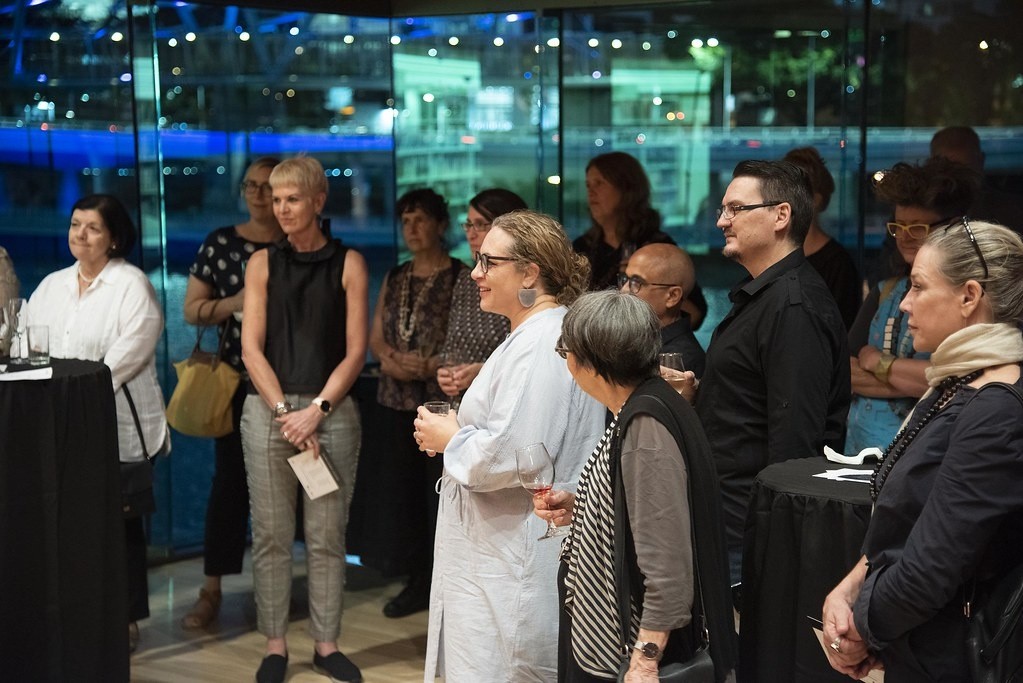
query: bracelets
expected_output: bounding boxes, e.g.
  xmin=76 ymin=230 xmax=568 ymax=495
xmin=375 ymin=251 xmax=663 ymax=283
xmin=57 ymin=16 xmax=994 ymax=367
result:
xmin=873 ymin=352 xmax=895 ymax=385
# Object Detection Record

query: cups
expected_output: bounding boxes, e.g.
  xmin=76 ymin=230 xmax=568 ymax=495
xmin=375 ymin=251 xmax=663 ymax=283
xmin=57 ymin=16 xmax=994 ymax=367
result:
xmin=423 ymin=401 xmax=450 ymax=451
xmin=658 ymin=352 xmax=687 ymax=394
xmin=26 ymin=325 xmax=50 ymax=366
xmin=0 ymin=307 xmax=9 ymax=339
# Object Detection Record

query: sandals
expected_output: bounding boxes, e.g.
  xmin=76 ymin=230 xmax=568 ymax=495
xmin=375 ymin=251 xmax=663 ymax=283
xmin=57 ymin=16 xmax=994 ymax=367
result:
xmin=182 ymin=587 xmax=222 ymax=630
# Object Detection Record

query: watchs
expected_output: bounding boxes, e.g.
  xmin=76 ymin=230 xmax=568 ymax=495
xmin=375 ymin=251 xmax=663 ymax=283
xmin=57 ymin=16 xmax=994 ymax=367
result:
xmin=311 ymin=396 xmax=334 ymax=416
xmin=274 ymin=400 xmax=293 ymax=417
xmin=633 ymin=641 xmax=664 ymax=661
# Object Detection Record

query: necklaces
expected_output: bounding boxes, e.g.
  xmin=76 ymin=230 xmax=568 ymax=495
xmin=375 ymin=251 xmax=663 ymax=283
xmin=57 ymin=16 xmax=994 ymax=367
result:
xmin=883 ymin=297 xmax=914 ymax=360
xmin=868 ymin=368 xmax=982 ymax=502
xmin=398 ymin=250 xmax=444 ymax=343
xmin=892 ymin=278 xmax=918 ymax=408
xmin=78 ymin=265 xmax=98 ymax=282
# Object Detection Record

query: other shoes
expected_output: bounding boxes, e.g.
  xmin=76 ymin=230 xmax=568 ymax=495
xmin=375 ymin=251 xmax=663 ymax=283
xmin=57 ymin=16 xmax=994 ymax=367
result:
xmin=256 ymin=651 xmax=289 ymax=683
xmin=383 ymin=584 xmax=429 ymax=618
xmin=312 ymin=649 xmax=363 ymax=682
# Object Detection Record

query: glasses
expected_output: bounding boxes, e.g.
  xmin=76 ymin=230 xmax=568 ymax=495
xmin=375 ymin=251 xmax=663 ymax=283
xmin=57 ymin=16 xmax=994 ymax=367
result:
xmin=616 ymin=272 xmax=677 ymax=294
xmin=716 ymin=203 xmax=778 ymax=221
xmin=475 ymin=250 xmax=518 ymax=275
xmin=886 ymin=219 xmax=947 ymax=239
xmin=555 ymin=334 xmax=571 ymax=360
xmin=460 ymin=222 xmax=492 ymax=233
xmin=945 ymin=216 xmax=988 ymax=297
xmin=241 ymin=179 xmax=272 ymax=197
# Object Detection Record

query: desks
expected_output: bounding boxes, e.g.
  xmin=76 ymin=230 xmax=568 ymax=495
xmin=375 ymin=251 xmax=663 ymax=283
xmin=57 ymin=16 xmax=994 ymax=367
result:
xmin=0 ymin=353 xmax=131 ymax=683
xmin=738 ymin=453 xmax=877 ymax=683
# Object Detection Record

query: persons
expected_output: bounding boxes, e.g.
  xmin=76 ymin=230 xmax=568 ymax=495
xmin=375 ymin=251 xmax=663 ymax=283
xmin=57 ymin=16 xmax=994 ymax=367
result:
xmin=0 ymin=120 xmax=1023 ymax=683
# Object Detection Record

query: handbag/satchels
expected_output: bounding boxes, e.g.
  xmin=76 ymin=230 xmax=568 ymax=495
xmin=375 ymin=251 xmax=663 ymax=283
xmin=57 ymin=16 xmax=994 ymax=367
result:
xmin=122 ymin=460 xmax=154 ymax=519
xmin=965 ymin=562 xmax=1023 ymax=683
xmin=617 ymin=649 xmax=718 ymax=682
xmin=164 ymin=297 xmax=239 ymax=436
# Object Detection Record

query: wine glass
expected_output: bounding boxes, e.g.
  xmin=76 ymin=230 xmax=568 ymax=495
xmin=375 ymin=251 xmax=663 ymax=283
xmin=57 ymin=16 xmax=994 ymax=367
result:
xmin=514 ymin=442 xmax=572 ymax=541
xmin=9 ymin=298 xmax=29 ymax=365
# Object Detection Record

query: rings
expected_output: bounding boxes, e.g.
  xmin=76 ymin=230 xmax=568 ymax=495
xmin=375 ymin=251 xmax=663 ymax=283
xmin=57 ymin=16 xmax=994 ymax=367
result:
xmin=830 ymin=635 xmax=846 ymax=652
xmin=283 ymin=432 xmax=290 ymax=439
